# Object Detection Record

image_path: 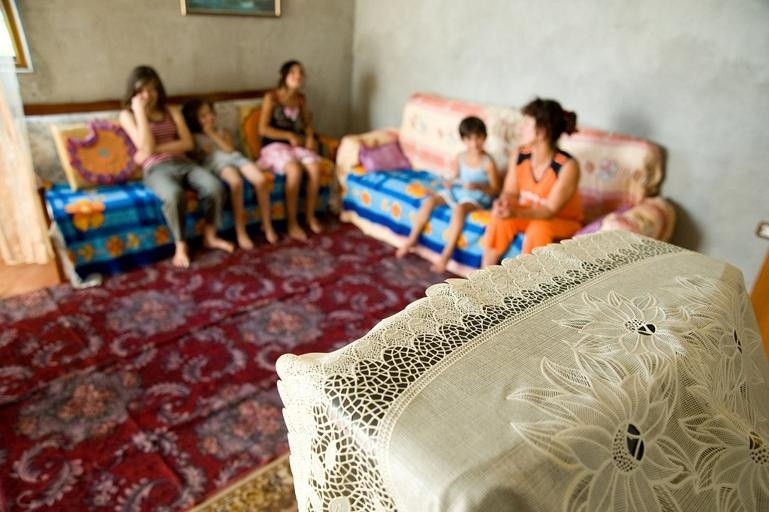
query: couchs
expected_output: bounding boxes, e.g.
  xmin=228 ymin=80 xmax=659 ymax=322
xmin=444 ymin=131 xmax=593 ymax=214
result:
xmin=334 ymin=92 xmax=680 ymax=274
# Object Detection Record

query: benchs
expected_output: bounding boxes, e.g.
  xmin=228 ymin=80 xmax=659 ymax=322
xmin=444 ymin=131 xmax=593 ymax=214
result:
xmin=13 ymin=87 xmax=335 ymax=278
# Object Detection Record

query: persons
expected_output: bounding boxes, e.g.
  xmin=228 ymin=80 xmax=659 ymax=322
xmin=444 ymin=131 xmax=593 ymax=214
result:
xmin=182 ymin=97 xmax=279 ymax=251
xmin=395 ymin=117 xmax=502 ymax=273
xmin=480 ymin=99 xmax=584 ymax=270
xmin=118 ymin=66 xmax=235 ymax=268
xmin=256 ymin=61 xmax=323 ymax=241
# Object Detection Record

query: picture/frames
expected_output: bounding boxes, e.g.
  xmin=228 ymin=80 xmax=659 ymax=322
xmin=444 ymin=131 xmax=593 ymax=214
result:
xmin=0 ymin=0 xmax=33 ymax=75
xmin=180 ymin=1 xmax=282 ymax=18
